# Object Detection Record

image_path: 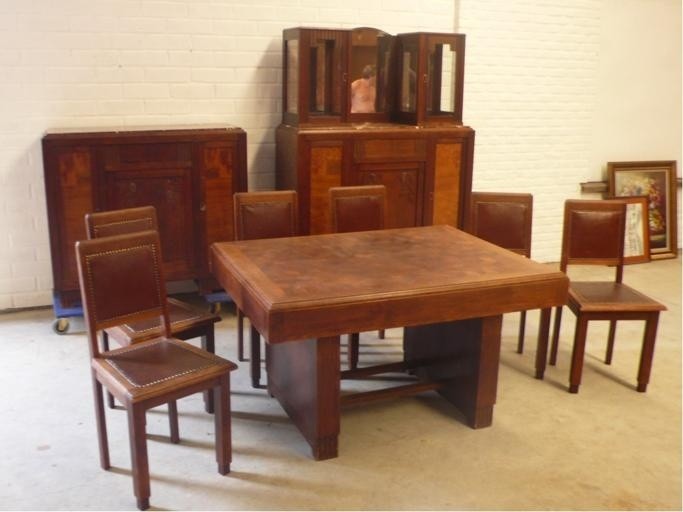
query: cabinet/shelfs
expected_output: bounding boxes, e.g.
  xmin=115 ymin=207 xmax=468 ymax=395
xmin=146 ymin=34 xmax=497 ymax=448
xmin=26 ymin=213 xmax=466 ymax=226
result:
xmin=38 ymin=126 xmax=251 ymax=308
xmin=274 ymin=25 xmax=479 ymax=237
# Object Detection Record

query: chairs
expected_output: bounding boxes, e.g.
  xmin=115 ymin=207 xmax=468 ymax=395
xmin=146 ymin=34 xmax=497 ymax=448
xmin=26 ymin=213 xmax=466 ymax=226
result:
xmin=72 ymin=230 xmax=239 ymax=510
xmin=84 ymin=204 xmax=223 ymax=414
xmin=466 ymin=192 xmax=552 ymax=381
xmin=549 ymin=198 xmax=668 ymax=393
xmin=231 ymin=181 xmax=393 ymax=388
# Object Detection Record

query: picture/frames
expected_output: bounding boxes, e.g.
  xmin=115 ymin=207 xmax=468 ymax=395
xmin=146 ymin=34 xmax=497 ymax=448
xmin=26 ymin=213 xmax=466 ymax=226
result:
xmin=605 ymin=160 xmax=678 ymax=261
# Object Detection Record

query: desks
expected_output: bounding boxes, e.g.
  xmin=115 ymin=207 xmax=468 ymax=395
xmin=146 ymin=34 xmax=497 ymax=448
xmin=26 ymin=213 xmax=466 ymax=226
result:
xmin=204 ymin=222 xmax=572 ymax=461
